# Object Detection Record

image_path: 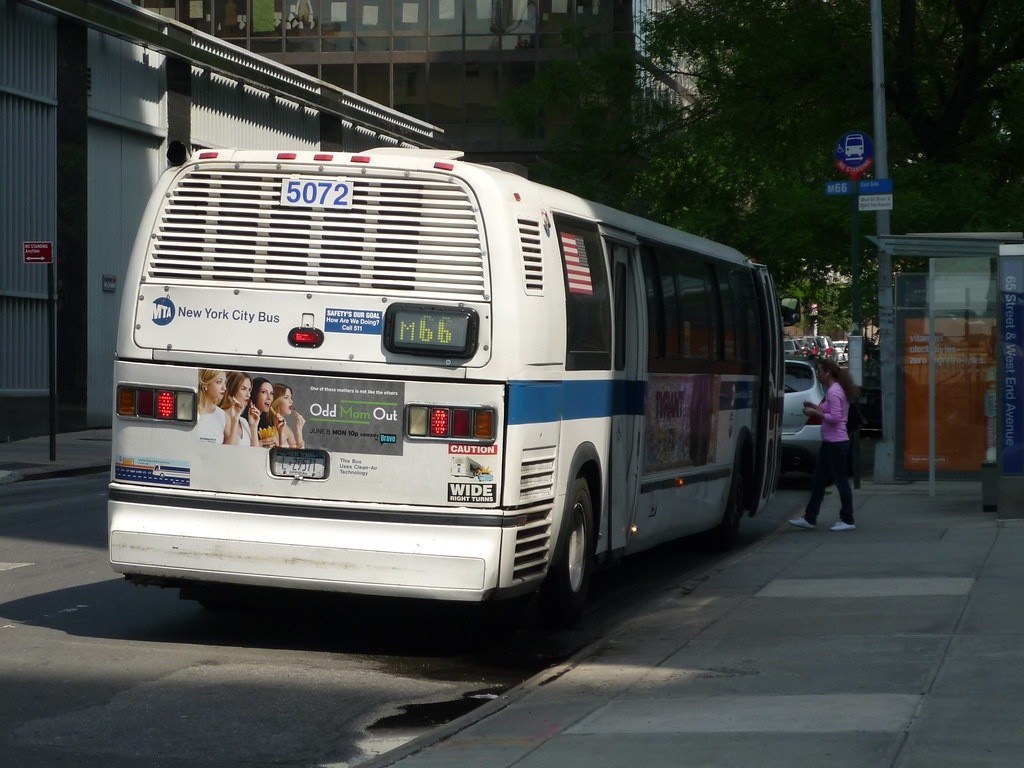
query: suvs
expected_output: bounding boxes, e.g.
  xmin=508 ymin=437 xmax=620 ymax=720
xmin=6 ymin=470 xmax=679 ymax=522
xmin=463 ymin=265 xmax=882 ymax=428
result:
xmin=777 ymin=356 xmax=826 ymax=480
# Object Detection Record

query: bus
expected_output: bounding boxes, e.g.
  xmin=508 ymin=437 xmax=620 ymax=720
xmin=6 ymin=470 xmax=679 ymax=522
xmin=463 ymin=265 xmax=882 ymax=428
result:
xmin=106 ymin=147 xmax=785 ymax=625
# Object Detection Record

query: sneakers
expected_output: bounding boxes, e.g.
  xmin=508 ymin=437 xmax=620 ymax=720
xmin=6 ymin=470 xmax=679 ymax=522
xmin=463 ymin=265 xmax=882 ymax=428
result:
xmin=830 ymin=522 xmax=857 ymax=531
xmin=789 ymin=517 xmax=814 ymax=528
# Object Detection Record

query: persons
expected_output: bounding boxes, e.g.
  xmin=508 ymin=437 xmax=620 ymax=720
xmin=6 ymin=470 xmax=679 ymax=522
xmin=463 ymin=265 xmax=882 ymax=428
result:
xmin=786 ymin=357 xmax=857 ymax=530
xmin=196 ymin=369 xmax=242 ymax=445
xmin=218 ymin=372 xmax=260 ymax=446
xmin=271 ymin=383 xmax=306 ymax=449
xmin=240 ymin=376 xmax=286 ymax=448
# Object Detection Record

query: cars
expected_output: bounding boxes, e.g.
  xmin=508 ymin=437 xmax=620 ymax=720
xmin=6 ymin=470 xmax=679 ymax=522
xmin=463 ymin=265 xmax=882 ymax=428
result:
xmin=782 ymin=336 xmax=850 ymax=365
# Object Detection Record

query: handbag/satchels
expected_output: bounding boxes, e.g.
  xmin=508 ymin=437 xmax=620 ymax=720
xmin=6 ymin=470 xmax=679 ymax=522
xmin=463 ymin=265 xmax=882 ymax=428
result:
xmin=846 ymin=400 xmax=864 ymax=433
